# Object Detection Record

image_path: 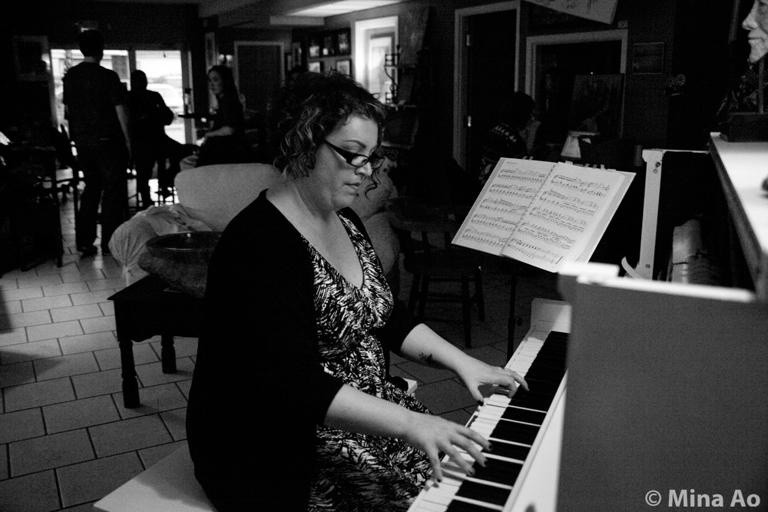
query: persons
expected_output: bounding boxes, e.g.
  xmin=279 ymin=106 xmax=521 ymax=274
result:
xmin=187 ymin=76 xmax=530 ymax=512
xmin=124 ymin=70 xmax=174 ymax=210
xmin=490 ymin=92 xmax=535 ymax=172
xmin=741 ymin=0 xmax=768 ymax=64
xmin=195 ymin=65 xmax=246 ymax=167
xmin=61 ymin=30 xmax=133 ymax=253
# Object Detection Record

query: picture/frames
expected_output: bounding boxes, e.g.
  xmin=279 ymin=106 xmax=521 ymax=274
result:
xmin=632 ymin=42 xmax=665 ymax=74
xmin=285 ymin=52 xmax=293 ymax=73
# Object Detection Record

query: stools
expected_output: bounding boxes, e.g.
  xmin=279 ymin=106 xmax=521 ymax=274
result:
xmin=93 ymin=444 xmax=224 ymax=512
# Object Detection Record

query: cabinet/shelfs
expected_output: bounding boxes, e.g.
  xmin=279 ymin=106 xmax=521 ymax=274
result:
xmin=308 ymin=27 xmax=352 ymax=77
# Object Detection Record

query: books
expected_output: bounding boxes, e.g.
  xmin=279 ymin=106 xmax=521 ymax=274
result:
xmin=450 ymin=157 xmax=638 ymax=277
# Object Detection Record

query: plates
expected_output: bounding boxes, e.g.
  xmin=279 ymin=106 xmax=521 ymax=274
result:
xmin=143 ymin=233 xmax=218 ymax=263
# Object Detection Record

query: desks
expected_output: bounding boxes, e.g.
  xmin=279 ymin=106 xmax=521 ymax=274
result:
xmin=107 ymin=275 xmax=205 ymax=409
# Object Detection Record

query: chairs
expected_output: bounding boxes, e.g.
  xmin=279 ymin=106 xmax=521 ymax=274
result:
xmin=390 ymin=160 xmax=487 ymax=348
xmin=110 ymin=162 xmax=398 ymax=288
xmin=55 ymin=124 xmax=87 ymax=203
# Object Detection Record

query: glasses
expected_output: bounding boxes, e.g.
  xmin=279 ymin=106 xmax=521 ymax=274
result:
xmin=325 ymin=139 xmax=384 ymax=170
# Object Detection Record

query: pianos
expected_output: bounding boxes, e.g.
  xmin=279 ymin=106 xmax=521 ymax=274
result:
xmin=409 ymin=132 xmax=768 ymax=512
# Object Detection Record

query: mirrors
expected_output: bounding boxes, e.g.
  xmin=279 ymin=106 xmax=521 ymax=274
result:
xmin=452 ymin=1 xmax=520 ymax=169
xmin=526 ymin=29 xmax=629 ymax=141
xmin=233 ymin=41 xmax=287 ymax=119
